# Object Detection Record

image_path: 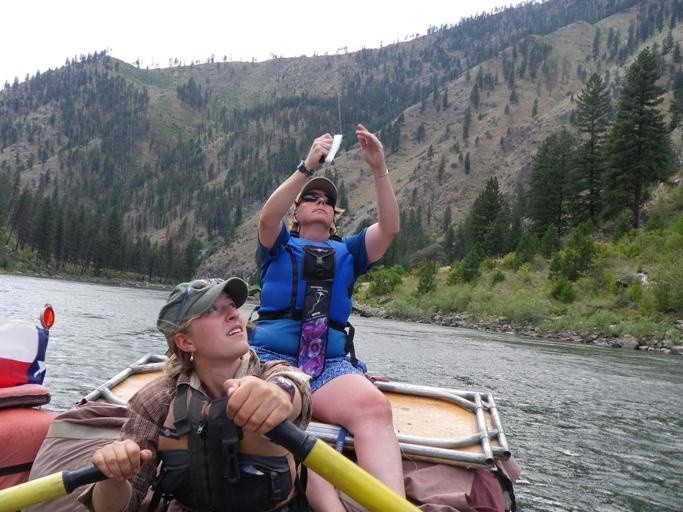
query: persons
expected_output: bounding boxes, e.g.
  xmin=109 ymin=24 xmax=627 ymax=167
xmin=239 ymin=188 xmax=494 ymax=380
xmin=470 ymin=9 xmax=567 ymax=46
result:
xmin=76 ymin=274 xmax=313 ymax=510
xmin=246 ymin=124 xmax=407 ymax=510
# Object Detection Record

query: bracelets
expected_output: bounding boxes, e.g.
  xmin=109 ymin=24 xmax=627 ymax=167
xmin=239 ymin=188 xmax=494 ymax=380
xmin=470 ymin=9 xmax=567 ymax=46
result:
xmin=374 ymin=169 xmax=390 ymax=177
xmin=296 ymin=160 xmax=314 ymax=176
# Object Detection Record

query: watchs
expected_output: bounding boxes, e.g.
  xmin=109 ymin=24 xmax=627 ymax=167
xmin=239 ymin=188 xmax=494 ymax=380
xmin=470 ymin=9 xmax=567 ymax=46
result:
xmin=269 ymin=376 xmax=294 ymax=403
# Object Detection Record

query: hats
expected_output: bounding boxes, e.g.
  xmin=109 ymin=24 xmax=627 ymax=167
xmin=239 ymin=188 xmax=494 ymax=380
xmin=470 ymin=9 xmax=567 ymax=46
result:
xmin=294 ymin=176 xmax=338 ymax=207
xmin=156 ymin=276 xmax=249 ymax=359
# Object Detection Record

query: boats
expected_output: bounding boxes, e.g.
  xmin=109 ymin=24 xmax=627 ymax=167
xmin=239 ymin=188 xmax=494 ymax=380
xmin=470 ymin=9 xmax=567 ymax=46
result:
xmin=27 ymin=350 xmax=526 ymax=512
xmin=0 ymin=302 xmax=69 ymax=512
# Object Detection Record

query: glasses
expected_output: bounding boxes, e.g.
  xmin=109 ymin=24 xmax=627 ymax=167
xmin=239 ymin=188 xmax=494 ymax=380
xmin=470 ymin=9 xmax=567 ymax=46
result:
xmin=174 ymin=277 xmax=226 ymax=325
xmin=300 ymin=191 xmax=336 ymax=207
